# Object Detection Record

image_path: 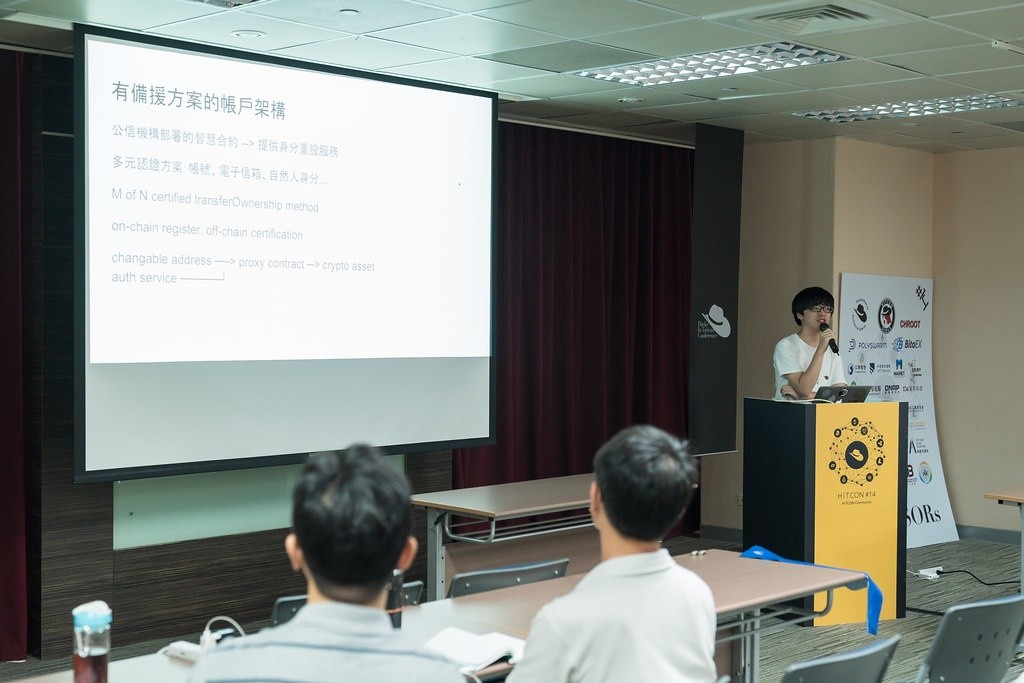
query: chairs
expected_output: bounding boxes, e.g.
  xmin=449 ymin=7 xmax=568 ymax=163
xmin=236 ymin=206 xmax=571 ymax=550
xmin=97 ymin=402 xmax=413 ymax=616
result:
xmin=446 ymin=557 xmax=571 ymax=600
xmin=782 ymin=633 xmax=902 ymax=683
xmin=270 ymin=580 xmax=424 ymax=626
xmin=913 ymin=594 xmax=1024 ymax=683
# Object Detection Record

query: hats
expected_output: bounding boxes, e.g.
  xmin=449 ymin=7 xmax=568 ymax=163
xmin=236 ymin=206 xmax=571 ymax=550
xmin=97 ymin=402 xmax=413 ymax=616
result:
xmin=854 ymin=304 xmax=867 ymax=322
xmin=701 ymin=304 xmax=731 ymax=338
xmin=881 ymin=305 xmax=891 ymax=316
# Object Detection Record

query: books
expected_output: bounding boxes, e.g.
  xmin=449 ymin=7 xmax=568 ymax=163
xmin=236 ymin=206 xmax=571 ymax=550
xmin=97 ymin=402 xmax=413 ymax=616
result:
xmin=421 ymin=629 xmax=526 ymax=670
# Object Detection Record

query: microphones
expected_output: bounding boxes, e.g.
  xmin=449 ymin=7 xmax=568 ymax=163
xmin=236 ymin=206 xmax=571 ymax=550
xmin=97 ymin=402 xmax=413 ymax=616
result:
xmin=819 ymin=323 xmax=840 ymax=356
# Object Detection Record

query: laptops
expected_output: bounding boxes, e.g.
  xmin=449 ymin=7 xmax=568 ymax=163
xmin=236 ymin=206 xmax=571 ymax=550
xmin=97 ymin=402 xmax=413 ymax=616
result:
xmin=812 ymin=386 xmax=872 ymax=404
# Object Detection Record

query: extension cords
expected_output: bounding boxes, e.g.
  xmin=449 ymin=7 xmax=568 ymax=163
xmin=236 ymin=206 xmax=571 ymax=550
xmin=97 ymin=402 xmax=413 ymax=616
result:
xmin=917 ymin=567 xmax=943 ymax=578
xmin=166 ymin=641 xmax=202 ymax=667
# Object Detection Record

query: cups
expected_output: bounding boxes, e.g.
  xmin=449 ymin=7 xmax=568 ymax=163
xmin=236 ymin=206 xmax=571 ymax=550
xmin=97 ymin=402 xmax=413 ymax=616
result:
xmin=72 ymin=604 xmax=112 ymax=683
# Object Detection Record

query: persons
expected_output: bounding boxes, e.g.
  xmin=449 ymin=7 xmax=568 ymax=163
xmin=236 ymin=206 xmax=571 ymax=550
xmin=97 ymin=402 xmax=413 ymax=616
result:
xmin=504 ymin=425 xmax=719 ymax=682
xmin=773 ymin=286 xmax=848 ymax=404
xmin=185 ymin=442 xmax=471 ymax=683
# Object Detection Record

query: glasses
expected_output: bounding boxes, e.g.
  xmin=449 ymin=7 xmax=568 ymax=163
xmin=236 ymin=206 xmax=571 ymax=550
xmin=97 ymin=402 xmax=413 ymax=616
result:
xmin=803 ymin=304 xmax=833 ymax=313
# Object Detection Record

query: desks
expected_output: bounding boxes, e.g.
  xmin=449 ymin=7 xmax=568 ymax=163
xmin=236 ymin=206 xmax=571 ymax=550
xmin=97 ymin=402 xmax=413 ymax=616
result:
xmin=0 ymin=549 xmax=865 ymax=683
xmin=411 ymin=473 xmax=593 ymax=600
xmin=983 ymin=486 xmax=1024 ymax=597
